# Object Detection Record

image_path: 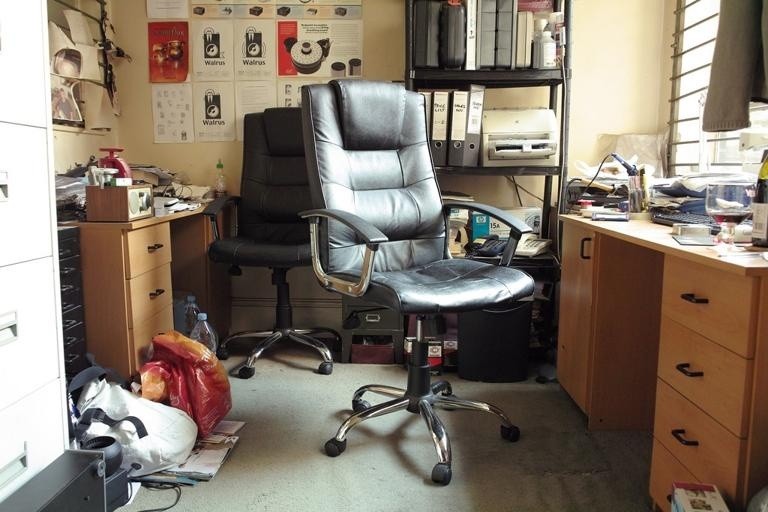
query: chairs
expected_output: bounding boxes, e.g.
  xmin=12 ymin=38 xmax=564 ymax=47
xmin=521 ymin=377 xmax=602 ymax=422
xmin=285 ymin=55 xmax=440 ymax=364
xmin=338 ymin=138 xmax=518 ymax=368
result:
xmin=209 ymin=106 xmax=344 ymax=379
xmin=298 ymin=77 xmax=538 ymax=488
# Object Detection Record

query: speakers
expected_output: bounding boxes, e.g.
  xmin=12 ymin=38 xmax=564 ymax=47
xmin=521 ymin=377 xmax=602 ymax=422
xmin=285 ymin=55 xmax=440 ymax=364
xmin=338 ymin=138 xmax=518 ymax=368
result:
xmin=86 ymin=185 xmax=153 ymax=222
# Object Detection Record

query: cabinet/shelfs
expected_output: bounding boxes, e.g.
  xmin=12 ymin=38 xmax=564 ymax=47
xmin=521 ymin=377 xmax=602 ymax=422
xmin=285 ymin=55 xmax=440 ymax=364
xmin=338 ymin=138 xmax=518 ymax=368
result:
xmin=0 ymin=0 xmax=106 ymax=510
xmin=553 ymin=213 xmax=768 ymax=509
xmin=402 ymin=2 xmax=573 ymax=349
xmin=58 ymin=226 xmax=86 ymax=380
xmin=63 ymin=203 xmax=232 ymax=383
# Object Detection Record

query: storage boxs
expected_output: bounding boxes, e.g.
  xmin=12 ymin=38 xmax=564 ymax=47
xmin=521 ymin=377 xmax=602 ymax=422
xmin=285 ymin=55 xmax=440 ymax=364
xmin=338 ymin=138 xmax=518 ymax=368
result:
xmin=349 ymin=343 xmax=395 ymax=366
xmin=83 ymin=183 xmax=158 ymax=224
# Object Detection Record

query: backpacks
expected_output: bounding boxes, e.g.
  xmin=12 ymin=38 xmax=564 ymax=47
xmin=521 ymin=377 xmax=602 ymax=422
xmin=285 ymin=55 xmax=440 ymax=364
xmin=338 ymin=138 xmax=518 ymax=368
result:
xmin=67 ymin=364 xmax=198 ymax=477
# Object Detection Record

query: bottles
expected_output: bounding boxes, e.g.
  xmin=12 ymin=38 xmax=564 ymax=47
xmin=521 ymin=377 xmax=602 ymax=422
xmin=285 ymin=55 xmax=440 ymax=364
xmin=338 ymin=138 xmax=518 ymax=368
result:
xmin=213 ymin=157 xmax=226 ymax=198
xmin=640 ymin=169 xmax=649 ymax=212
xmin=189 ymin=313 xmax=217 ymax=357
xmin=531 ymin=15 xmax=565 ymax=70
xmin=183 ymin=295 xmax=201 ymax=335
xmin=752 ymin=149 xmax=768 ymax=248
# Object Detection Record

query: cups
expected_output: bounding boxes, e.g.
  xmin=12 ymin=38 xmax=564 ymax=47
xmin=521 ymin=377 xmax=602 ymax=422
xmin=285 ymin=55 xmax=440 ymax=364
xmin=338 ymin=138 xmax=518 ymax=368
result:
xmin=628 ymin=175 xmax=643 ymax=212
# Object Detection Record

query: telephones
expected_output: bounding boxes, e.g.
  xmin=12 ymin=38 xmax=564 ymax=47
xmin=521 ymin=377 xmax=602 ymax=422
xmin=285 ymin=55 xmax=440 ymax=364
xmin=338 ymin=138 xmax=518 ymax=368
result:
xmin=464 ymin=235 xmax=553 ymax=256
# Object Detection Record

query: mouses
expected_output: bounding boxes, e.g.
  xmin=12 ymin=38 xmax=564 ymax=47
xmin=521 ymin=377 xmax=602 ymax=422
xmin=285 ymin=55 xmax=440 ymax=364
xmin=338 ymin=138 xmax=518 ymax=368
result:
xmin=721 ymin=225 xmax=752 ymax=243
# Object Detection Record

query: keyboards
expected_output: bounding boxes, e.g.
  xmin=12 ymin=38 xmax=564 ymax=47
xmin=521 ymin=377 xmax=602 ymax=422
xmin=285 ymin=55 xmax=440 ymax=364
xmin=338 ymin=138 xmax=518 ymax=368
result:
xmin=652 ymin=212 xmax=753 ymax=236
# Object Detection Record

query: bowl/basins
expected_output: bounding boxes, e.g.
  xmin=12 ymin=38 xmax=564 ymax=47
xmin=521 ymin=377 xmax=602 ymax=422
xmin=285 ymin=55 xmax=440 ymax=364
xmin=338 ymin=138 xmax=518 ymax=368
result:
xmin=83 ymin=435 xmax=123 ymax=478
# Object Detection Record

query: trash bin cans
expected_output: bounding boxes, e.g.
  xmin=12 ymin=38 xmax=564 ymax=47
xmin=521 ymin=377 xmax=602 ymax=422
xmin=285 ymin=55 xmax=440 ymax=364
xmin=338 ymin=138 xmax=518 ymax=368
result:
xmin=457 ymin=301 xmax=533 ymax=384
xmin=672 ymin=481 xmax=730 ymax=512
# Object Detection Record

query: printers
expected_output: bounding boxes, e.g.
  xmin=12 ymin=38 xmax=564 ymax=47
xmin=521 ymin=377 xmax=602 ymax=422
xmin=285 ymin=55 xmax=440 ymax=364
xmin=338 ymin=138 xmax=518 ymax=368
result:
xmin=479 ymin=107 xmax=560 ymax=167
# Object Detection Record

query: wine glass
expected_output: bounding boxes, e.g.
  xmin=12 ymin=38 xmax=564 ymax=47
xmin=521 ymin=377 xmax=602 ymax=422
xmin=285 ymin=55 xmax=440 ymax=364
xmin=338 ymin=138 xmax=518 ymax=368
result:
xmin=704 ymin=180 xmax=752 ymax=253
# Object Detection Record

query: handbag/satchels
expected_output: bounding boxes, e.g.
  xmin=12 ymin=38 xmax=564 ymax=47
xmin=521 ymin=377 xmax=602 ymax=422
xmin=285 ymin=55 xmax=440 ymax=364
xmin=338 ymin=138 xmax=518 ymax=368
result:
xmin=130 ymin=328 xmax=232 ymax=439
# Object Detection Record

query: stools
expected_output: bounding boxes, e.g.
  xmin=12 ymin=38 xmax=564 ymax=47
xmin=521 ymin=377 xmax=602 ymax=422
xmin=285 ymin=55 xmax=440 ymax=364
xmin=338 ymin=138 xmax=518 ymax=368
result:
xmin=337 ymin=287 xmax=405 ymax=365
